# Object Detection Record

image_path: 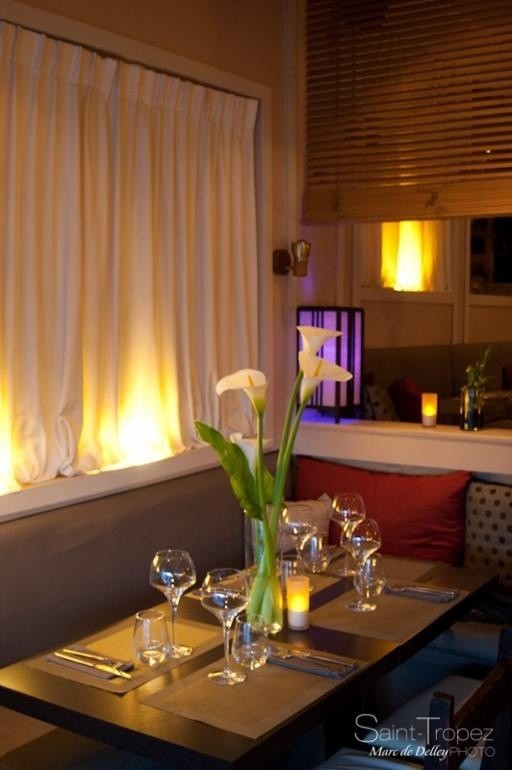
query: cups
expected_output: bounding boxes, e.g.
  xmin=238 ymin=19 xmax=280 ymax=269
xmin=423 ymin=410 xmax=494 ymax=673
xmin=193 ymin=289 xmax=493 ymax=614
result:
xmin=458 ymin=385 xmax=485 ymax=431
xmin=301 ymin=531 xmax=329 ymax=574
xmin=353 ymin=552 xmax=386 ymax=597
xmin=132 ymin=609 xmax=170 ymax=667
xmin=230 ymin=613 xmax=271 ymax=671
xmin=274 ymin=555 xmax=306 ymax=600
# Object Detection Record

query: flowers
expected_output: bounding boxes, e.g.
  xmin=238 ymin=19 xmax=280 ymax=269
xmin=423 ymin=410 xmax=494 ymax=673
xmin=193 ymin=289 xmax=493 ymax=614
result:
xmin=192 ymin=321 xmax=354 ymax=632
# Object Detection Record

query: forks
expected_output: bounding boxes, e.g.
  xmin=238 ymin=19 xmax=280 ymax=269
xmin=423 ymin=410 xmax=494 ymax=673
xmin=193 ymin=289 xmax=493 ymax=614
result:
xmin=62 ymin=647 xmax=131 ymax=669
xmin=270 ymin=645 xmax=348 ymax=673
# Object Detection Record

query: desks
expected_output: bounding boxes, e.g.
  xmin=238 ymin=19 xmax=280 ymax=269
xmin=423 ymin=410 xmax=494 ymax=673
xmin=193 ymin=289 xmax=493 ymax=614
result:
xmin=1 ymin=550 xmax=499 ymax=768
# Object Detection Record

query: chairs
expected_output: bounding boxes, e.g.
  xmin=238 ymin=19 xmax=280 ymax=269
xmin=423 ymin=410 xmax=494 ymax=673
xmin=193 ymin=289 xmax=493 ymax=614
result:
xmin=317 ymin=614 xmax=511 ymax=767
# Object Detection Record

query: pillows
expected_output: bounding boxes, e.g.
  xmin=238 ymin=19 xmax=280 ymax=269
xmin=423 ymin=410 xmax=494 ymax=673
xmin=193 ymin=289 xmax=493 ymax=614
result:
xmin=292 ymin=454 xmax=512 ymax=602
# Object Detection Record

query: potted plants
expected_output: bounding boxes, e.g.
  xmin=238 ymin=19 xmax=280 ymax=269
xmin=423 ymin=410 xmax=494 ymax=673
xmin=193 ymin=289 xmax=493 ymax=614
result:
xmin=456 ymin=343 xmax=493 ymax=430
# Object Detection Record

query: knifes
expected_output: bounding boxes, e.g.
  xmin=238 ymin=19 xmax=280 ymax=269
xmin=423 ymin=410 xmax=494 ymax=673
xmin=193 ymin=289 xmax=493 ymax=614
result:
xmin=54 ymin=652 xmax=132 ymax=680
xmin=290 ymin=648 xmax=355 ymax=668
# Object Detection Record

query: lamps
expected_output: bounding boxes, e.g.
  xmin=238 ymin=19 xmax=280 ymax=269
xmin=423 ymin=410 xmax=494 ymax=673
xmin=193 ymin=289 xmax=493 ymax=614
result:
xmin=273 ymin=239 xmax=311 ymax=277
xmin=295 ymin=305 xmax=365 ymax=424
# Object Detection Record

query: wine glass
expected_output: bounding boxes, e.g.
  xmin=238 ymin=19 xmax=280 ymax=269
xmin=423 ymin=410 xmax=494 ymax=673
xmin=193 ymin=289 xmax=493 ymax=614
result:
xmin=148 ymin=549 xmax=197 ymax=660
xmin=277 ymin=503 xmax=319 ymax=570
xmin=199 ymin=567 xmax=251 ymax=686
xmin=339 ymin=517 xmax=382 ymax=613
xmin=327 ymin=491 xmax=367 ymax=578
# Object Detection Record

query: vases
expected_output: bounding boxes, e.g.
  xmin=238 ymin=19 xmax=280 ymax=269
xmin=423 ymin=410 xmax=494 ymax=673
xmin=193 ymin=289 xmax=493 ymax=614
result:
xmin=245 ymin=517 xmax=284 ymax=634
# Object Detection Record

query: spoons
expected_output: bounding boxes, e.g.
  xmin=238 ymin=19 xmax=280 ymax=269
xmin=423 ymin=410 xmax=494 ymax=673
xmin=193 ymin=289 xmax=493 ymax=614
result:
xmin=387 ymin=582 xmax=451 ymax=599
